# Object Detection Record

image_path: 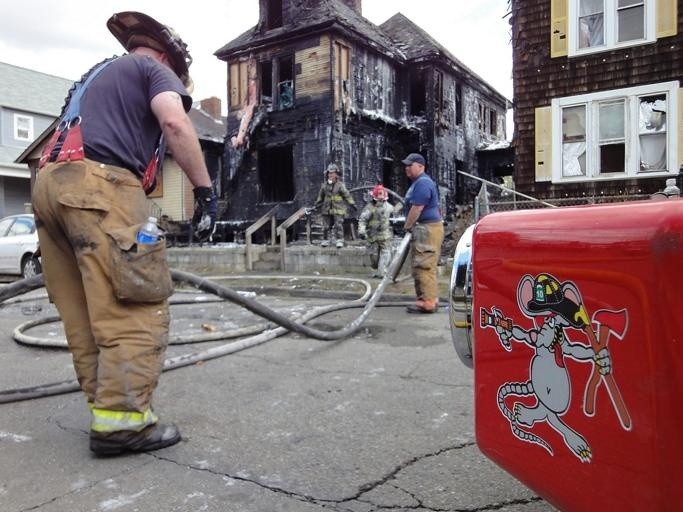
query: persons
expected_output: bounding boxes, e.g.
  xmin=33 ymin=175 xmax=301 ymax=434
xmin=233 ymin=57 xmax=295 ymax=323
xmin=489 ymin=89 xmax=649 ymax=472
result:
xmin=30 ymin=10 xmax=219 ymax=456
xmin=315 ymin=163 xmax=356 ymax=247
xmin=395 ymin=153 xmax=445 ymax=315
xmin=358 ymin=183 xmax=398 ymax=279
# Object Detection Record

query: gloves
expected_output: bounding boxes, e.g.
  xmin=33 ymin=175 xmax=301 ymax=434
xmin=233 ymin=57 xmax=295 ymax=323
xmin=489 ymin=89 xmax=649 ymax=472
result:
xmin=189 ymin=186 xmax=218 ymax=242
xmin=348 ymin=204 xmax=357 ymax=212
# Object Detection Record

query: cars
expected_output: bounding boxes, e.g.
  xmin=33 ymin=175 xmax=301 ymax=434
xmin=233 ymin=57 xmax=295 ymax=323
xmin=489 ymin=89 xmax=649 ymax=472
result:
xmin=440 ymin=196 xmax=682 ymax=512
xmin=1 ymin=206 xmax=49 ymax=283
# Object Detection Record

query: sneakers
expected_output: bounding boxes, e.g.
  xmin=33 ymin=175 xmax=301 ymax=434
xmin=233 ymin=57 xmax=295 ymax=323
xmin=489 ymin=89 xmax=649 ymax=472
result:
xmin=319 ymin=238 xmax=344 ymax=249
xmin=405 ymin=297 xmax=440 ymax=315
xmin=85 ymin=395 xmax=182 ymax=459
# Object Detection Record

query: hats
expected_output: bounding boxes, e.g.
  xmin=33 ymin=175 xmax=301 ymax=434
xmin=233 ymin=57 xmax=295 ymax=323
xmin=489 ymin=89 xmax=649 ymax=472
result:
xmin=367 ymin=184 xmax=390 ymax=202
xmin=322 ymin=163 xmax=340 ymax=175
xmin=96 ymin=9 xmax=199 ymax=98
xmin=400 ymin=152 xmax=426 ymax=167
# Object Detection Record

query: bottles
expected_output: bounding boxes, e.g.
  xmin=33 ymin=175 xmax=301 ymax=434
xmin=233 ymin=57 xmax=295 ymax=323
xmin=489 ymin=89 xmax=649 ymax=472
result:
xmin=138 ymin=216 xmax=158 ymax=242
xmin=664 ymin=177 xmax=681 ymax=195
